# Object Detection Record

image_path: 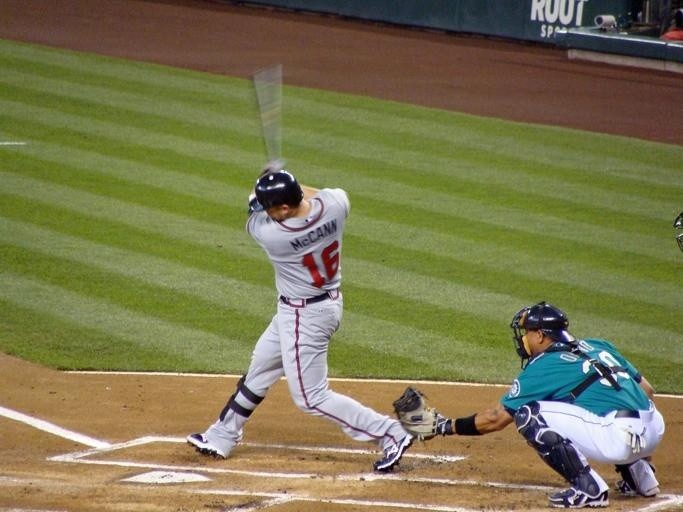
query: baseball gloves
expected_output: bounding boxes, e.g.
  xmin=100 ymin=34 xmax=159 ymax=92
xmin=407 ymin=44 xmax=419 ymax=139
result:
xmin=394 ymin=388 xmax=447 ymax=441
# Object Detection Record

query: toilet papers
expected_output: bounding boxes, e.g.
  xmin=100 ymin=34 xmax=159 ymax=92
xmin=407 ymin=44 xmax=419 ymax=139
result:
xmin=593 ymin=15 xmax=616 ymax=28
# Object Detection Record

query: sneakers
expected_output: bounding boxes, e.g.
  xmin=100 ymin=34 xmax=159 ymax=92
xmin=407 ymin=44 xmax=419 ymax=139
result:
xmin=186 ymin=433 xmax=231 ymax=460
xmin=546 ymin=486 xmax=610 ymax=508
xmin=372 ymin=431 xmax=415 ymax=470
xmin=615 ymin=477 xmax=657 ymax=498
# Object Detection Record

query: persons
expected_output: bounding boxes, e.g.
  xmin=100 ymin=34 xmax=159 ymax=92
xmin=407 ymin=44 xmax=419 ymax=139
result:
xmin=673 ymin=212 xmax=683 ymax=251
xmin=186 ymin=170 xmax=417 ymax=472
xmin=395 ymin=302 xmax=666 ymax=510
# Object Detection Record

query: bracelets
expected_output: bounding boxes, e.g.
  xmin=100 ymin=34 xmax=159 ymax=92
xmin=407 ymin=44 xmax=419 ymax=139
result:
xmin=454 ymin=413 xmax=483 ymax=437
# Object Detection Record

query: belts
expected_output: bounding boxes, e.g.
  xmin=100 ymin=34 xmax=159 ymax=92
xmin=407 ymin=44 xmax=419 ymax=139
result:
xmin=614 ymin=408 xmax=640 ymax=419
xmin=279 ymin=291 xmax=329 ymax=305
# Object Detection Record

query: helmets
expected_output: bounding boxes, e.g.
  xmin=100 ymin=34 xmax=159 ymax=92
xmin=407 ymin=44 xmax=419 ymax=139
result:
xmin=254 ymin=170 xmax=304 ymax=210
xmin=510 ymin=301 xmax=569 ymax=359
